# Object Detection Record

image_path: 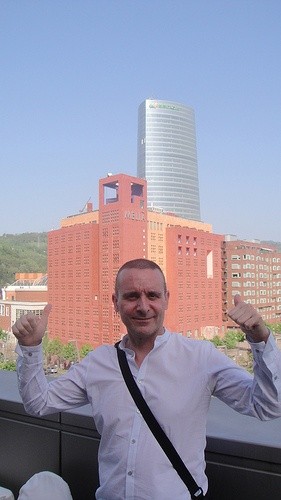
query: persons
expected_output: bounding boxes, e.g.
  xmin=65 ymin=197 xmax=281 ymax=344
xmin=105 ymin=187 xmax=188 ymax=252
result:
xmin=11 ymin=257 xmax=281 ymax=500
